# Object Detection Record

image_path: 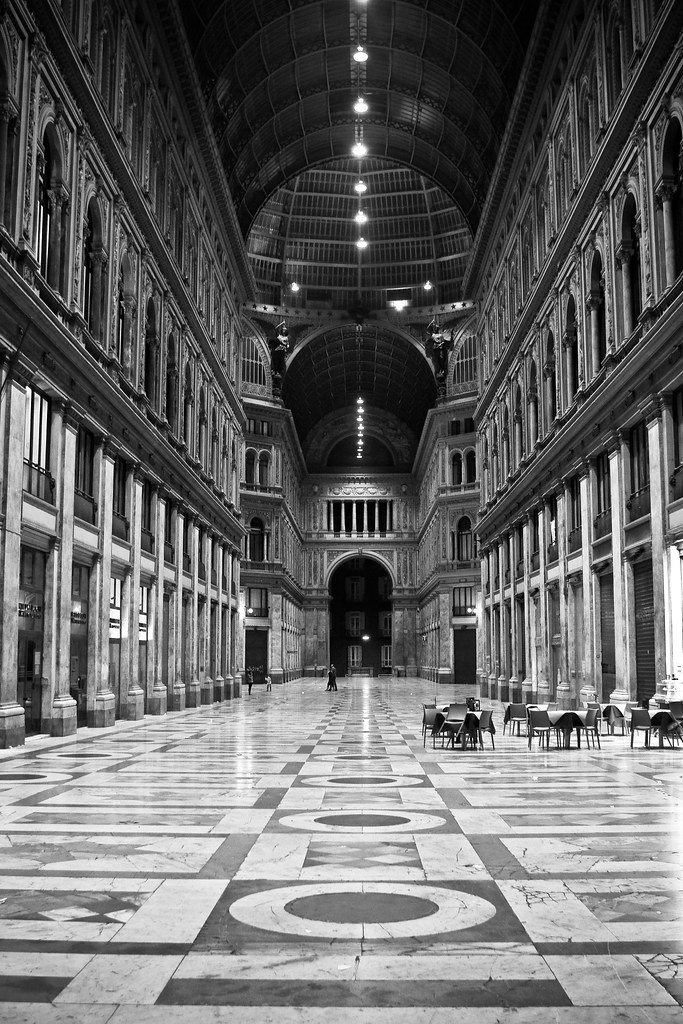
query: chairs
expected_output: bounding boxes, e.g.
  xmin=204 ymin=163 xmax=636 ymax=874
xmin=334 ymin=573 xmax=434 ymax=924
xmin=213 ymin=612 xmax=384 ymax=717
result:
xmin=581 ymin=701 xmax=640 ymax=736
xmin=631 ymin=701 xmax=683 ymax=750
xmin=421 ymin=703 xmax=496 ymax=751
xmin=502 ymin=702 xmax=559 ymax=737
xmin=526 ymin=704 xmax=602 ymax=751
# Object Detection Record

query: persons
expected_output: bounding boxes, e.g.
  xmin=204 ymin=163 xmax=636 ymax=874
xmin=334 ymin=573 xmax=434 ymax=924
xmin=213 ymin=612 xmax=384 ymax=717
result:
xmin=248 ymin=670 xmax=253 ymax=695
xmin=270 ymin=320 xmax=289 ymax=377
xmin=325 ymin=664 xmax=337 ymax=691
xmin=265 ymin=674 xmax=271 ymax=691
xmin=426 ymin=320 xmax=446 ymax=375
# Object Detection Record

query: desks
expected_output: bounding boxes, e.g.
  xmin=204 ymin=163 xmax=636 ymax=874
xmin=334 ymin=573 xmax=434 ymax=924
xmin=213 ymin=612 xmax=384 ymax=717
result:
xmin=440 ymin=711 xmax=481 ymax=744
xmin=546 ymin=710 xmax=587 ymax=751
xmin=647 ymin=709 xmax=676 ymax=749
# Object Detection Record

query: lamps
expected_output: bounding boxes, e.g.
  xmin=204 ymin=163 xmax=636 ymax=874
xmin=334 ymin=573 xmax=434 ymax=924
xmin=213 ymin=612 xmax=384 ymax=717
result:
xmin=353 ymin=45 xmax=368 ymax=62
xmin=349 ymin=142 xmax=368 ymax=158
xmin=354 ymin=208 xmax=369 ymax=226
xmin=357 ymin=431 xmax=363 ymax=437
xmin=357 ymin=406 xmax=364 ymax=414
xmin=357 ymin=452 xmax=363 ymax=459
xmin=356 ymin=237 xmax=368 ymax=250
xmin=354 ymin=98 xmax=368 ymax=113
xmin=357 ymin=438 xmax=364 ymax=445
xmin=357 ymin=424 xmax=364 ymax=431
xmin=356 ymin=415 xmax=364 ymax=422
xmin=354 ymin=180 xmax=368 ymax=193
xmin=356 ymin=396 xmax=365 ymax=404
xmin=356 ymin=446 xmax=363 ymax=452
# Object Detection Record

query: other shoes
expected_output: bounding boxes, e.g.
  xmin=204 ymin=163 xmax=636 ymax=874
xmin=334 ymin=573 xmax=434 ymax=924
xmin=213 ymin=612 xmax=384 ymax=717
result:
xmin=335 ymin=689 xmax=337 ymax=691
xmin=325 ymin=689 xmax=328 ymax=691
xmin=328 ymin=689 xmax=331 ymax=691
